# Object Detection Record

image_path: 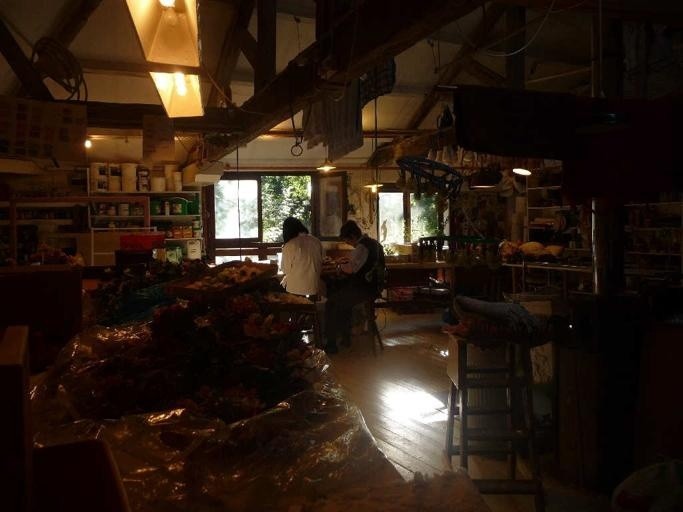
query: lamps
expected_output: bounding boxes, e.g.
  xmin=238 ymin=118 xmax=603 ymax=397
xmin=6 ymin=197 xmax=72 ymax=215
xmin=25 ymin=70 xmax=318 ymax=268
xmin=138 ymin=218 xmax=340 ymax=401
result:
xmin=143 ymin=66 xmax=205 ymax=121
xmin=470 ymin=155 xmax=495 ymax=188
xmin=316 ymin=146 xmax=336 ymax=173
xmin=512 ymin=159 xmax=534 ymax=177
xmin=364 ymin=137 xmax=384 ymax=194
xmin=122 ymin=0 xmax=204 ymax=70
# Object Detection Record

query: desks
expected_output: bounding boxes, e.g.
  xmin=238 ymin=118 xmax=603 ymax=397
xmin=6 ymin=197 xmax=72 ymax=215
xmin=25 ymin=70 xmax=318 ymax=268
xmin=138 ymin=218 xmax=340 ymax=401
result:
xmin=504 ymin=262 xmax=593 ymax=303
xmin=375 ymin=262 xmax=462 ymax=309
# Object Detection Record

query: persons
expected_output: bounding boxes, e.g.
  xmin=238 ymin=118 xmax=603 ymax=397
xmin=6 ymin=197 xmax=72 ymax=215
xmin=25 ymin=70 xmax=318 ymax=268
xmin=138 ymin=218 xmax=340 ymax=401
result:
xmin=323 ymin=220 xmax=384 ymax=354
xmin=281 ymin=217 xmax=326 ymax=332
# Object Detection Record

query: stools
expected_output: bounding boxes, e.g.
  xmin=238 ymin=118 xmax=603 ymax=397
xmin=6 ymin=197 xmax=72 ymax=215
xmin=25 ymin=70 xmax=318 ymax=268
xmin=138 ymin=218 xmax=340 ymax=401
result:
xmin=298 ymin=295 xmax=322 ymax=348
xmin=444 ymin=332 xmax=545 ymax=512
xmin=345 ymin=290 xmax=384 ymax=358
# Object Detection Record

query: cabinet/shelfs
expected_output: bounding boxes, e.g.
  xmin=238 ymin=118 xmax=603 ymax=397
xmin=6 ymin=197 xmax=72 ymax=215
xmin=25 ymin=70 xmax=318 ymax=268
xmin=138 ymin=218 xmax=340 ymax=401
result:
xmin=526 ymin=168 xmax=594 ymax=300
xmin=82 ymin=167 xmax=208 ymax=266
xmin=623 ymin=192 xmax=683 ymax=294
xmin=0 ymin=200 xmax=89 ymax=267
